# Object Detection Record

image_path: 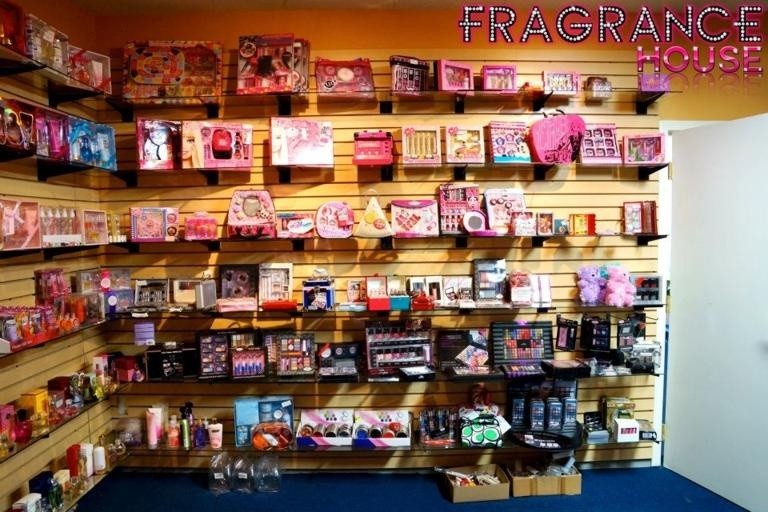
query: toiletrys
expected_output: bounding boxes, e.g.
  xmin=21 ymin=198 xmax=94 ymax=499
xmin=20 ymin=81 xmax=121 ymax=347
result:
xmin=112 ymin=402 xmax=223 ymax=451
xmin=0 ymin=370 xmax=122 ymax=512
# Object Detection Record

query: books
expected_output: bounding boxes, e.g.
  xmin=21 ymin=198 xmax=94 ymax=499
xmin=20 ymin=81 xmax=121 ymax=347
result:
xmin=1 ymin=1 xmax=117 ymax=248
xmin=124 ymin=31 xmax=667 ymax=246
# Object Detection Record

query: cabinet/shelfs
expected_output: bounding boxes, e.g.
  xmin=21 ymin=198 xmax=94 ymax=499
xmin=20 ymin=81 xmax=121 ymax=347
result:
xmin=99 ymin=46 xmax=669 ymax=470
xmin=0 ymin=27 xmax=111 ymax=512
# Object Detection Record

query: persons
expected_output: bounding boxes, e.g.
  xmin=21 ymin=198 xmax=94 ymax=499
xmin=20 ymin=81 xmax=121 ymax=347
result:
xmin=2 ymin=0 xmax=668 ymax=248
